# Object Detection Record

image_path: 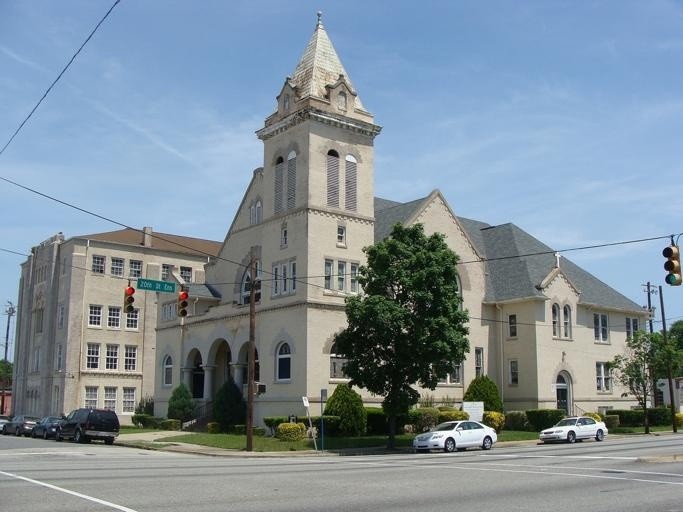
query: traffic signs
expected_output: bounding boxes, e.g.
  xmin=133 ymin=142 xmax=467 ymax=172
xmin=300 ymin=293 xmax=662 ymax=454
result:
xmin=137 ymin=277 xmax=176 ymax=293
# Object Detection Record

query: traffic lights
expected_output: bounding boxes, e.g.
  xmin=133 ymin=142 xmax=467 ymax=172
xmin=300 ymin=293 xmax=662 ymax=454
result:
xmin=124 ymin=287 xmax=135 ymax=313
xmin=255 ymin=382 xmax=266 ymax=396
xmin=177 ymin=292 xmax=189 ymax=317
xmin=662 ymin=245 xmax=682 ymax=286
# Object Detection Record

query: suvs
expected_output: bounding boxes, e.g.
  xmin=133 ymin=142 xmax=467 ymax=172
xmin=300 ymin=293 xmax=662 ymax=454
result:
xmin=56 ymin=408 xmax=120 ymax=445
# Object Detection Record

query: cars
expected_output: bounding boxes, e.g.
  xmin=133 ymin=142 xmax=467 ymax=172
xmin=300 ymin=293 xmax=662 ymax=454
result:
xmin=31 ymin=416 xmax=62 ymax=440
xmin=413 ymin=420 xmax=498 ymax=453
xmin=539 ymin=416 xmax=608 ymax=443
xmin=0 ymin=415 xmax=11 ymax=434
xmin=2 ymin=415 xmax=43 ymax=437
